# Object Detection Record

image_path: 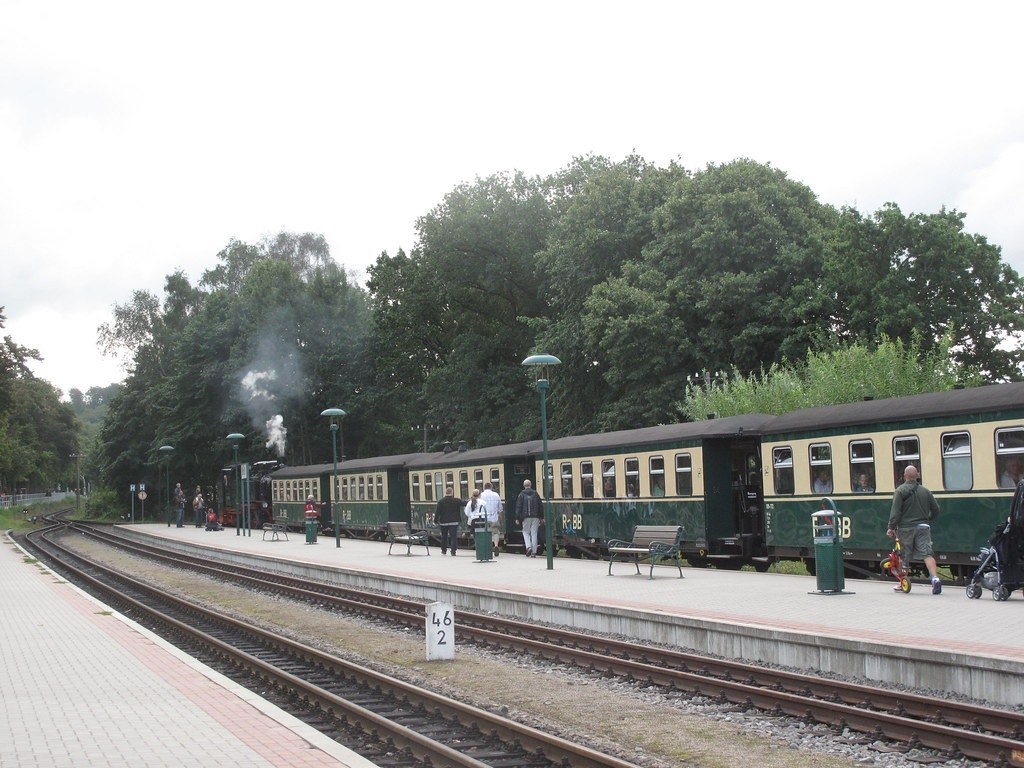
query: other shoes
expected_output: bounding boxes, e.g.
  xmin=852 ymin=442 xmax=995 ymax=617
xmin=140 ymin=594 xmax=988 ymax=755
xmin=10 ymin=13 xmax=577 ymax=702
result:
xmin=933 ymin=581 xmax=940 ymax=593
xmin=451 ymin=552 xmax=456 ymax=556
xmin=441 ymin=550 xmax=445 ymax=554
xmin=527 ymin=548 xmax=536 ymax=558
xmin=495 ymin=546 xmax=499 ymax=556
xmin=894 ymin=586 xmax=902 ymax=591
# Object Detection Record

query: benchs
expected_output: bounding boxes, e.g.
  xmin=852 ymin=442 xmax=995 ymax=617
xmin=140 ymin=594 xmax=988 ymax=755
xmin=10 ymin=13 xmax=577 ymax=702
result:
xmin=262 ymin=516 xmax=290 ymax=541
xmin=606 ymin=524 xmax=687 ymax=580
xmin=386 ymin=521 xmax=430 ymax=557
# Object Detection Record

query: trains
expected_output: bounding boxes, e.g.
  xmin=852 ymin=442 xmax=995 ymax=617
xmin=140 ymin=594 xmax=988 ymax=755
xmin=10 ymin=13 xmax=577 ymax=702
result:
xmin=221 ymin=380 xmax=1024 ymax=581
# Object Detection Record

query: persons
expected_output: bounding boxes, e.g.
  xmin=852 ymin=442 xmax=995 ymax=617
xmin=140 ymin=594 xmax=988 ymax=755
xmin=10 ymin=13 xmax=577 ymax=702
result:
xmin=856 ymin=473 xmax=873 ymax=492
xmin=174 ymin=482 xmax=219 ymax=531
xmin=480 ymin=482 xmax=503 ymax=555
xmin=433 ymin=487 xmax=468 ymax=555
xmin=514 ymin=479 xmax=544 ymax=557
xmin=304 ymin=495 xmax=326 ymax=525
xmin=887 ymin=466 xmax=941 ymax=595
xmin=997 ymin=457 xmax=1022 ymax=487
xmin=813 ymin=468 xmax=832 ymax=493
xmin=464 ymin=488 xmax=487 ymax=539
xmin=547 ymin=476 xmax=665 ymax=498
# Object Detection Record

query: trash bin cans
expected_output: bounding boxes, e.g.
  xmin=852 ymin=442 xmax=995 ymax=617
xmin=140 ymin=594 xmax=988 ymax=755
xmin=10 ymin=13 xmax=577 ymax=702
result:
xmin=305 ymin=504 xmax=318 ymax=544
xmin=811 ymin=498 xmax=846 ymax=592
xmin=473 ymin=504 xmax=494 ymax=561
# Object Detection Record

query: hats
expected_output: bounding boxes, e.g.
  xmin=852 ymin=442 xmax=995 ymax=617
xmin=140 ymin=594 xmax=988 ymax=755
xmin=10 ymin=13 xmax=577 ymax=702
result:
xmin=446 ymin=487 xmax=453 ymax=497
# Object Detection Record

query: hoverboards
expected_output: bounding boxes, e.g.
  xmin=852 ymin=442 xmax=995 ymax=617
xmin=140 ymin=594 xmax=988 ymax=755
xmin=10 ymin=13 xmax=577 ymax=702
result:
xmin=882 ymin=529 xmax=911 ymax=593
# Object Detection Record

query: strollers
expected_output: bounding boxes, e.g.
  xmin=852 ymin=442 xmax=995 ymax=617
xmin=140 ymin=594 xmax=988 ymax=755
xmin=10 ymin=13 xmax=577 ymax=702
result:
xmin=205 ymin=511 xmax=224 ymax=531
xmin=966 ymin=479 xmax=1024 ymax=601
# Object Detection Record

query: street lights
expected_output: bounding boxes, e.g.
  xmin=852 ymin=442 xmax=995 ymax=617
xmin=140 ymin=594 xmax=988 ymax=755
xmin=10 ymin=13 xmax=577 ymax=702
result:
xmin=159 ymin=446 xmax=175 ymax=527
xmin=320 ymin=408 xmax=347 ymax=547
xmin=226 ymin=434 xmax=246 ymax=536
xmin=521 ymin=355 xmax=562 ymax=570
